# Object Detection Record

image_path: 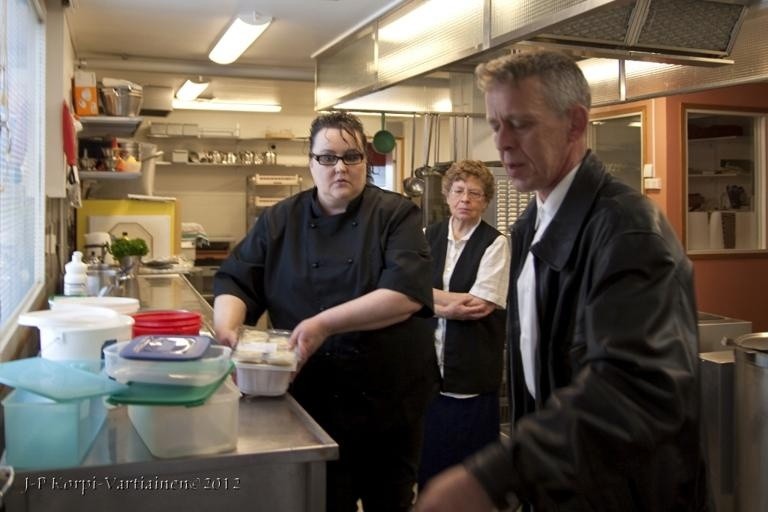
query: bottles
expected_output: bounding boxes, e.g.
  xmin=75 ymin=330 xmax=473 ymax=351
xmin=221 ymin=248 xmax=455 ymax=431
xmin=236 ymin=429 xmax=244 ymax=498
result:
xmin=63 ymin=251 xmax=89 ymax=297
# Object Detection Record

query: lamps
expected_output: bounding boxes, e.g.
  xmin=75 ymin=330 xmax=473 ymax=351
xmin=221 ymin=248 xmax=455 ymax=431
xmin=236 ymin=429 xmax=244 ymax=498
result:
xmin=208 ymin=12 xmax=275 ymax=65
xmin=175 ymin=78 xmax=211 ymax=101
xmin=172 ymin=99 xmax=283 ymax=114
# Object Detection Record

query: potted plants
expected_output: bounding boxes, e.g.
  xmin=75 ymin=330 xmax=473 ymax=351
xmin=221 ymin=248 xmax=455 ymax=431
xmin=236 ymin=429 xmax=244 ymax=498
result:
xmin=108 ymin=236 xmax=147 ymax=280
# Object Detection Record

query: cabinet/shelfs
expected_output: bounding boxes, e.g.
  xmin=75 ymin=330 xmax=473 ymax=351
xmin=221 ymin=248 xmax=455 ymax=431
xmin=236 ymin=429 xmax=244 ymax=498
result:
xmin=147 ymin=131 xmax=311 ymax=167
xmin=245 ymin=173 xmax=304 ymax=237
xmin=688 ymin=129 xmax=754 ymax=217
xmin=70 ymin=113 xmax=149 ymax=183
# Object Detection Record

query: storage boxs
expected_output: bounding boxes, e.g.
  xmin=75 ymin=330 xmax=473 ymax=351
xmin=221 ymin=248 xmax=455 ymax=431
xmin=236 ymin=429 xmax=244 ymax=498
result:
xmin=73 ymin=70 xmax=100 ymax=117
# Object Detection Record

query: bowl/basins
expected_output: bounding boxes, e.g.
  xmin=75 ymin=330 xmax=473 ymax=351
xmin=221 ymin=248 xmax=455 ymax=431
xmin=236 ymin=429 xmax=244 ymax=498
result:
xmin=207 ymin=148 xmax=277 ymax=166
xmin=101 ymin=141 xmax=138 ymax=172
xmin=101 ymin=86 xmax=145 ymax=116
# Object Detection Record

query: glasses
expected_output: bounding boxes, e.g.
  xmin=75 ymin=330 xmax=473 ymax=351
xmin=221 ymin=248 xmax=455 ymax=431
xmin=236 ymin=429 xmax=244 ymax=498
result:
xmin=313 ymin=151 xmax=366 ymax=165
xmin=451 ymin=187 xmax=486 ymax=200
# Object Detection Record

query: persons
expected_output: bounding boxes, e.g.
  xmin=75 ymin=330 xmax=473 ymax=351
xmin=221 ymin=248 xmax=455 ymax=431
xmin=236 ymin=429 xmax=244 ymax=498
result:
xmin=410 ymin=45 xmax=708 ymax=512
xmin=210 ymin=107 xmax=442 ymax=512
xmin=414 ymin=157 xmax=514 ymax=498
